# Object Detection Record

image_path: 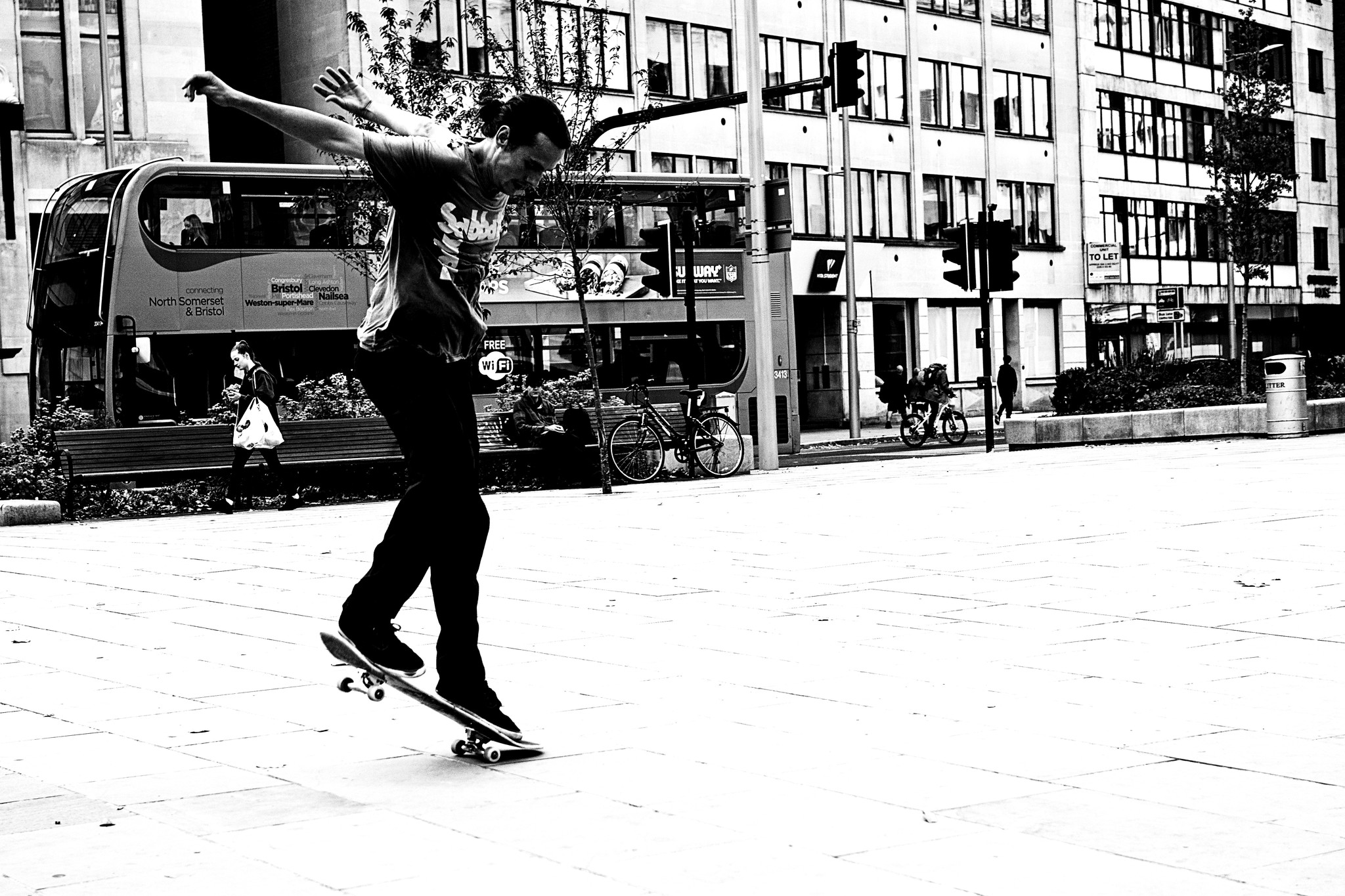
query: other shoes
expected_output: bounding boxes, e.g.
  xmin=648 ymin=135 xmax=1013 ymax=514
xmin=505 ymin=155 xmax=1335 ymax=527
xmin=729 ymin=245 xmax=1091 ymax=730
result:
xmin=885 ymin=423 xmax=892 ymax=428
xmin=904 ymin=420 xmax=914 ymax=428
xmin=925 ymin=428 xmax=936 ymax=439
xmin=995 ymin=412 xmax=1002 ymax=425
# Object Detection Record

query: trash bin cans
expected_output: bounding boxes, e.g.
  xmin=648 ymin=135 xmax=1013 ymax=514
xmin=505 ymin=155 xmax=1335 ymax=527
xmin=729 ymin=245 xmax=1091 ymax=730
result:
xmin=1263 ymin=354 xmax=1311 ymax=439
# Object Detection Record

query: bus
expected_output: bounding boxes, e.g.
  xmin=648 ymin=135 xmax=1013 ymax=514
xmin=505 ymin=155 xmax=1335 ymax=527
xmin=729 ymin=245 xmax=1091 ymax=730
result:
xmin=28 ymin=156 xmax=802 ymax=457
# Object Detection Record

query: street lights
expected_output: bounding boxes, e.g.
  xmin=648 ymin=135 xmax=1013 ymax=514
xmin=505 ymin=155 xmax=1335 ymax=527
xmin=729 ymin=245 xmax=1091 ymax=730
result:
xmin=1220 ymin=42 xmax=1284 ymax=359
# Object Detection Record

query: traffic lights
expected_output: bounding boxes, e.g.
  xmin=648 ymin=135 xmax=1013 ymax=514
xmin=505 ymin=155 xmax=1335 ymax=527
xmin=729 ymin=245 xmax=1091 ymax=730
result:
xmin=942 ymin=221 xmax=973 ymax=292
xmin=975 ymin=328 xmax=992 ymax=349
xmin=835 ymin=39 xmax=865 ymax=110
xmin=637 ymin=222 xmax=675 ymax=299
xmin=993 ymin=219 xmax=1021 ymax=292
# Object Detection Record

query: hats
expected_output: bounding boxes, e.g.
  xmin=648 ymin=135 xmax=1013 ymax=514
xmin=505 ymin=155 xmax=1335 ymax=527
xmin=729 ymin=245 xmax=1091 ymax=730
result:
xmin=525 ymin=372 xmax=543 ymax=384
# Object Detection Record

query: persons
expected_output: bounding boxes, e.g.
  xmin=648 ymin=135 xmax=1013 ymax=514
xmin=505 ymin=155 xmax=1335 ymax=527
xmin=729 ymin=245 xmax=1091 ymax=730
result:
xmin=878 ymin=365 xmax=938 ymax=429
xmin=206 ymin=340 xmax=305 ymax=515
xmin=994 ymin=355 xmax=1018 ymax=425
xmin=916 ymin=356 xmax=956 ymax=439
xmin=182 ymin=65 xmax=573 ymax=737
xmin=183 ymin=214 xmax=209 ymax=248
xmin=513 ymin=370 xmax=588 ymax=491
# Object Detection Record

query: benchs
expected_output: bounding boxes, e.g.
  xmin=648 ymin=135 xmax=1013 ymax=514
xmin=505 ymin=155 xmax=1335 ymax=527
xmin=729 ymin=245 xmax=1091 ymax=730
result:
xmin=53 ymin=403 xmax=687 ymax=521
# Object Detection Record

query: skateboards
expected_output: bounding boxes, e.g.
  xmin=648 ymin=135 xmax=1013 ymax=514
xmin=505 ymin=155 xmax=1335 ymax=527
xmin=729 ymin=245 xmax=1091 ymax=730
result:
xmin=319 ymin=632 xmax=543 ymax=763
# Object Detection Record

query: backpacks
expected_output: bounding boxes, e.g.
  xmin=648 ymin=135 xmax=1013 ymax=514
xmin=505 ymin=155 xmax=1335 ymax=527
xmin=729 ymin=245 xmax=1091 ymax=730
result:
xmin=875 ymin=379 xmax=890 ymax=404
xmin=916 ymin=367 xmax=940 ymax=392
xmin=562 ymin=400 xmax=599 ymax=445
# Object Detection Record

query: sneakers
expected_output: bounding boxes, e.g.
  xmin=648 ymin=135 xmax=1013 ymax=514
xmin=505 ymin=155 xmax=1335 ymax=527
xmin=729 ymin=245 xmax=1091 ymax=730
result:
xmin=338 ymin=609 xmax=426 ymax=678
xmin=207 ymin=498 xmax=234 ymax=514
xmin=277 ymin=492 xmax=306 ymax=511
xmin=435 ymin=678 xmax=523 ymax=740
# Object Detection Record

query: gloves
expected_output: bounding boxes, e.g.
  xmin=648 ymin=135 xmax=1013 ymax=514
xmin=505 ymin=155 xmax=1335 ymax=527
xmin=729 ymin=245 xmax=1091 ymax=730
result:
xmin=949 ymin=392 xmax=956 ymax=398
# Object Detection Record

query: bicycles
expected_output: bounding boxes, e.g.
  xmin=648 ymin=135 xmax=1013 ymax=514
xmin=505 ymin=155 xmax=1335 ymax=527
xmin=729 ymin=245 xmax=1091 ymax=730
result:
xmin=604 ymin=375 xmax=740 ymax=484
xmin=899 ymin=394 xmax=968 ymax=449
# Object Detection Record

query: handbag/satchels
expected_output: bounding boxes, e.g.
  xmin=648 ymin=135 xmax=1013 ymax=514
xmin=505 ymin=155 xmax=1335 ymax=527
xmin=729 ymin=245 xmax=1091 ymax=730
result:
xmin=254 ymin=397 xmax=285 ymax=449
xmin=233 ymin=397 xmax=266 ymax=450
